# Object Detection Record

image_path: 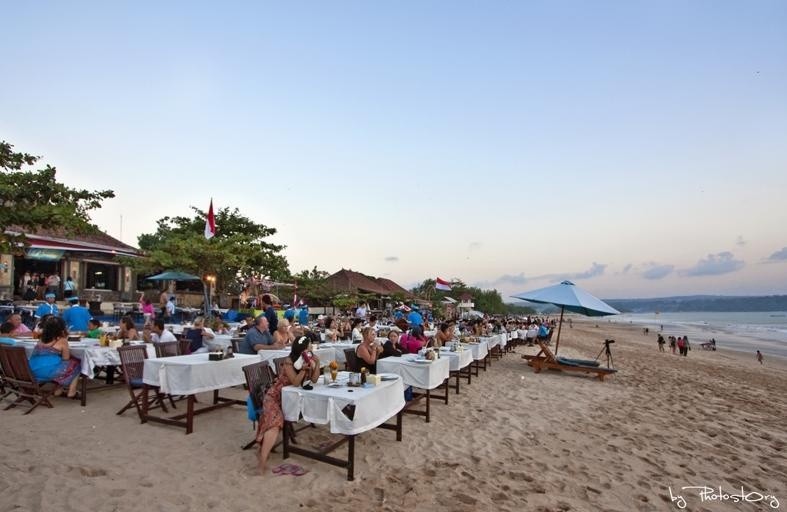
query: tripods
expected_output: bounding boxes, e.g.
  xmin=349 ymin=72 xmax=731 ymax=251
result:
xmin=584 ymin=345 xmax=617 ymax=377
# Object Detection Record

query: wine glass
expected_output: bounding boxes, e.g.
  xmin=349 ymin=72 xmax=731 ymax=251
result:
xmin=329 ymin=364 xmax=341 ymax=384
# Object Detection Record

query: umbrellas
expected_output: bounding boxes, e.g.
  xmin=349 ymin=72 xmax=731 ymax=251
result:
xmin=510 ymin=279 xmax=621 ymax=355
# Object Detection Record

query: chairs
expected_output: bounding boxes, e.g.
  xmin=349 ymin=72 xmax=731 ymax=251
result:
xmin=273 ymin=358 xmax=318 ymax=432
xmin=230 ymin=337 xmax=244 ymax=353
xmin=5 ymin=344 xmax=64 ymax=414
xmin=116 ymin=344 xmax=169 ymax=423
xmin=154 ymin=342 xmax=198 ymax=413
xmin=344 ymin=348 xmax=357 ymax=370
xmin=125 ymin=302 xmax=134 ymax=324
xmin=113 ymin=302 xmax=123 ymax=324
xmin=1 ymin=344 xmax=8 ymax=412
xmin=381 ymin=342 xmax=384 ymax=347
xmin=242 ymin=360 xmax=297 ymax=454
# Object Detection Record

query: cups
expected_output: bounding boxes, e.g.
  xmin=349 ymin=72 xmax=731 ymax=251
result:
xmin=352 ymin=373 xmax=360 ymax=385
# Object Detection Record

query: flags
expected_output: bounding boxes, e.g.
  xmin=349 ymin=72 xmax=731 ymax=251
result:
xmin=204 ymin=197 xmax=215 ymax=240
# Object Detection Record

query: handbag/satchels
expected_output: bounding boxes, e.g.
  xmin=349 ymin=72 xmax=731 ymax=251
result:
xmin=253 ymin=382 xmax=272 ymax=408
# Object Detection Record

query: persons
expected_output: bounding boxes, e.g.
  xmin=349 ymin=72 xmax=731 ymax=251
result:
xmin=1 ymin=271 xmax=556 ymax=397
xmin=710 ymin=338 xmax=716 ymax=351
xmin=251 ymin=336 xmax=320 ymax=477
xmin=756 ymin=349 xmax=763 ymax=364
xmin=656 ymin=333 xmax=691 ymax=357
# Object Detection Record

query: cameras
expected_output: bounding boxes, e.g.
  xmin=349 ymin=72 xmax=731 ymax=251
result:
xmin=603 ymin=339 xmax=615 ymax=345
